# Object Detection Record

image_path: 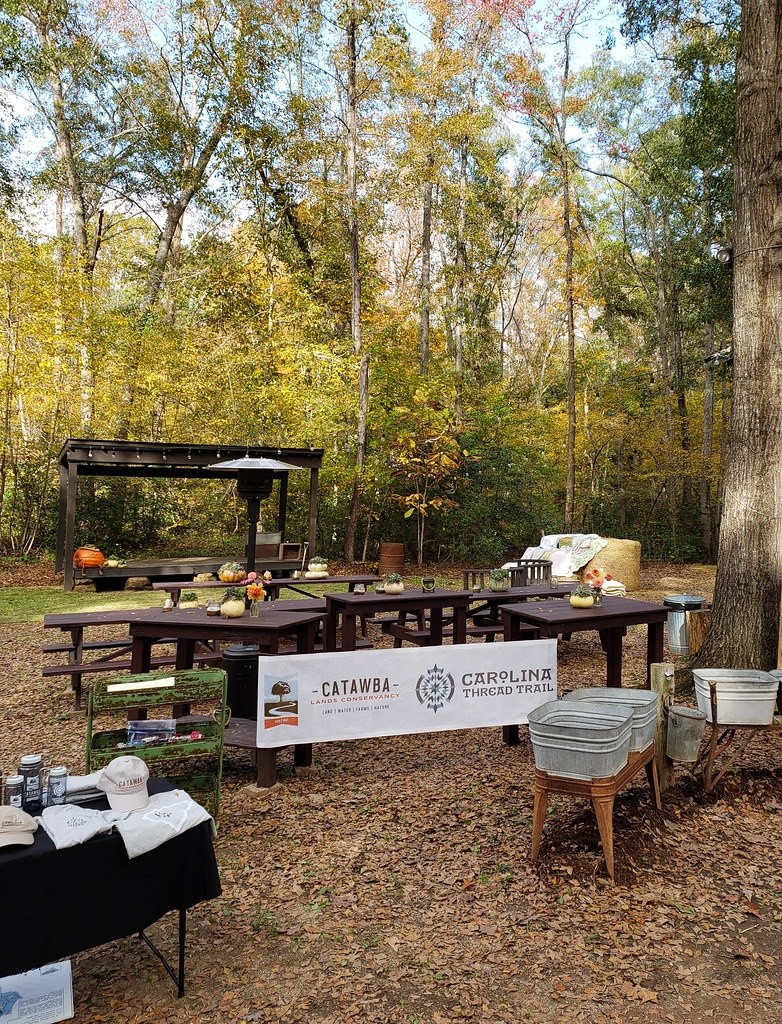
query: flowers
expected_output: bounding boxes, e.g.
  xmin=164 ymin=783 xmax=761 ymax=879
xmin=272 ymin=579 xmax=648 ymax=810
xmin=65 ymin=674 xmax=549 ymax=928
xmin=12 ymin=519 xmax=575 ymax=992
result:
xmin=585 ymin=568 xmax=613 ymax=590
xmin=245 ymin=582 xmax=267 ymax=604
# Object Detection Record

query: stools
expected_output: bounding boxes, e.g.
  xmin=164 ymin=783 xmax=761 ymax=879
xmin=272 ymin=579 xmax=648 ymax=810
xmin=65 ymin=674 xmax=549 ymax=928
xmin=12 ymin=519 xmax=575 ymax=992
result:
xmin=530 ymin=743 xmax=662 ymax=880
xmin=689 ymin=713 xmax=782 ymax=793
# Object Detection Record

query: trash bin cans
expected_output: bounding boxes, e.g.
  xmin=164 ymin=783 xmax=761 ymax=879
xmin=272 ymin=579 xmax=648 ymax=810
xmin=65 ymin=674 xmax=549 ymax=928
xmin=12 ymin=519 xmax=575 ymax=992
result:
xmin=665 ymin=596 xmax=706 ymax=655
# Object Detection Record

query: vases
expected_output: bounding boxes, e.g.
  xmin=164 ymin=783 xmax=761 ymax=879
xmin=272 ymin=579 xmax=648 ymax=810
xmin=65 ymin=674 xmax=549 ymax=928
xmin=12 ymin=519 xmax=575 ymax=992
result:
xmin=592 ymin=592 xmax=604 ymax=607
xmin=249 ymin=599 xmax=259 ymax=618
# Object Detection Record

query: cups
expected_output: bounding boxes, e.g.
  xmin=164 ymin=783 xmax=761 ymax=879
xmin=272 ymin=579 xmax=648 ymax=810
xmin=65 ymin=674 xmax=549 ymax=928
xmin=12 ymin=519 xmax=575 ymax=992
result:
xmin=421 ymin=577 xmax=435 ymax=593
xmin=550 ymin=575 xmax=558 ymax=589
xmin=164 ymin=598 xmax=174 ymax=607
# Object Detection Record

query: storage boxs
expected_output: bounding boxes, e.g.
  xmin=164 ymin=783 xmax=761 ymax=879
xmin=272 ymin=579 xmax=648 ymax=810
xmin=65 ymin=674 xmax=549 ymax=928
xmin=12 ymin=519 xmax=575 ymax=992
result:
xmin=0 ymin=960 xmax=74 ymax=1024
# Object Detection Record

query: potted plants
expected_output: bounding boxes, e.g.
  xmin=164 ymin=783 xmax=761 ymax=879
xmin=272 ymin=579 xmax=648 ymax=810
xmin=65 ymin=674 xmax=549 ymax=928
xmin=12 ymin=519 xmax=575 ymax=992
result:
xmin=178 ymin=592 xmax=199 ymax=610
xmin=488 ymin=568 xmax=512 ymax=592
xmin=570 ymin=582 xmax=594 ymax=608
xmin=107 ymin=554 xmax=119 ymax=568
xmin=219 ymin=586 xmax=246 ymax=618
xmin=217 ymin=560 xmax=247 ymax=583
xmin=308 ymin=557 xmax=329 ymax=572
xmin=382 ymin=572 xmax=405 ymax=594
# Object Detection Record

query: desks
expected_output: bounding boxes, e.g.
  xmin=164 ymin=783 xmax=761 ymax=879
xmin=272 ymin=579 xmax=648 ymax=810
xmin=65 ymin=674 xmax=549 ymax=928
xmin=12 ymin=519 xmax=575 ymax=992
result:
xmin=460 ymin=584 xmax=593 ymax=642
xmin=267 ymin=574 xmax=384 ymax=638
xmin=503 ymin=596 xmax=672 ymax=746
xmin=152 ymin=581 xmax=270 ymax=608
xmin=0 ymin=777 xmax=224 ymax=998
xmin=124 ymin=608 xmax=328 ymax=786
xmin=260 ymin=599 xmax=326 ymax=643
xmin=43 ymin=605 xmax=206 ymax=710
xmin=323 ymin=588 xmax=474 ymax=652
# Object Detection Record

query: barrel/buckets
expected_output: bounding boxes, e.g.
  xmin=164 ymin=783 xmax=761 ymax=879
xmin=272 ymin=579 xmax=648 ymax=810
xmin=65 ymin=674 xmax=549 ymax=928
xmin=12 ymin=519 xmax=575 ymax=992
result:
xmin=379 ymin=543 xmax=404 ymax=576
xmin=662 ymin=693 xmax=708 ymax=762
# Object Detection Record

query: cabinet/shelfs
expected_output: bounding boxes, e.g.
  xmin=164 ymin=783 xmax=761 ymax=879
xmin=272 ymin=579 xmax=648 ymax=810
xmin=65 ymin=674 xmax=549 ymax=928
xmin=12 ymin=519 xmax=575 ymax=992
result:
xmin=84 ymin=668 xmax=229 ymax=825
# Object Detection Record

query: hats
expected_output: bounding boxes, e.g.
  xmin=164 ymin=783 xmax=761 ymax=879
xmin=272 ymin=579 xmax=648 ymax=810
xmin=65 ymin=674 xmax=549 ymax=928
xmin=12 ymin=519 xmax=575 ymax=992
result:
xmin=0 ymin=806 xmax=38 ymax=847
xmin=95 ymin=755 xmax=149 ymax=813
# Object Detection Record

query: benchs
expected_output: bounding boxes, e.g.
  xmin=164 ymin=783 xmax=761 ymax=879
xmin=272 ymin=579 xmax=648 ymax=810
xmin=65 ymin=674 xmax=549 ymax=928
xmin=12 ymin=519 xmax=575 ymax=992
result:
xmin=43 ymin=650 xmax=223 ymax=692
xmin=405 ymin=622 xmax=541 ymax=647
xmin=43 ymin=637 xmax=179 ymax=656
xmin=364 ymin=609 xmax=502 ymax=636
xmin=278 ymin=639 xmax=374 ymax=655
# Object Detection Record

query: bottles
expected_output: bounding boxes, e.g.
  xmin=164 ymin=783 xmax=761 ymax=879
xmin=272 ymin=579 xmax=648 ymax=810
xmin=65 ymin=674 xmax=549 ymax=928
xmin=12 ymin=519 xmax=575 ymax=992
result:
xmin=4 ymin=775 xmax=25 ymax=810
xmin=39 ymin=766 xmax=67 ymax=807
xmin=19 ymin=754 xmax=43 ymax=808
xmin=473 ymin=585 xmax=481 ymax=593
